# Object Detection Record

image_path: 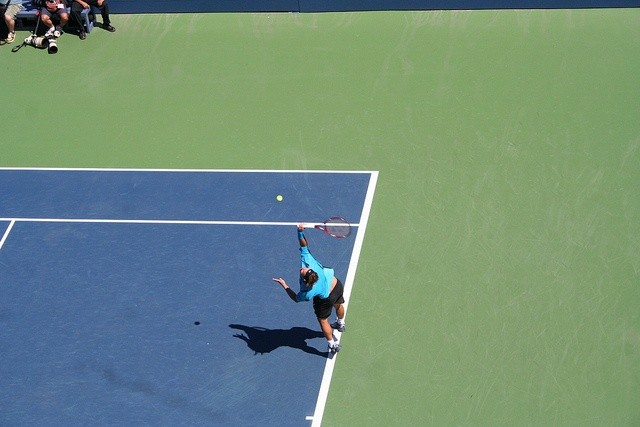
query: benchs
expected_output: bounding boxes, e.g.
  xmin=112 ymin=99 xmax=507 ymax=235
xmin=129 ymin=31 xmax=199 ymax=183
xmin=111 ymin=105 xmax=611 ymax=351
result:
xmin=1 ymin=0 xmax=94 ymax=32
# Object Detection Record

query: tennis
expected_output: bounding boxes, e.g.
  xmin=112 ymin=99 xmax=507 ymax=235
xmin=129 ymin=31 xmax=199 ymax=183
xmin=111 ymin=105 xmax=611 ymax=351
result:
xmin=277 ymin=194 xmax=283 ymax=201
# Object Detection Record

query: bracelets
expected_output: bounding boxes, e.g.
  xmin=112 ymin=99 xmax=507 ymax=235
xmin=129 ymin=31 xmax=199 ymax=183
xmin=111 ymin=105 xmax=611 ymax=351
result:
xmin=297 ymin=230 xmax=306 ymax=240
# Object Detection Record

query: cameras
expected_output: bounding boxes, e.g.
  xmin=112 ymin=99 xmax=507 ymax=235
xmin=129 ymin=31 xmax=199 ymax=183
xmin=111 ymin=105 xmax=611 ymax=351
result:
xmin=43 ymin=29 xmax=64 ymax=54
xmin=24 ymin=35 xmax=48 ymax=49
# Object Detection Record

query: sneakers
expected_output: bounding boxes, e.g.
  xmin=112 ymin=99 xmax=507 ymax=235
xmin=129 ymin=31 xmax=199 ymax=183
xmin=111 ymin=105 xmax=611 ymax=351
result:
xmin=330 ymin=322 xmax=344 ymax=332
xmin=45 ymin=31 xmax=53 ymax=37
xmin=7 ymin=31 xmax=15 ymax=44
xmin=103 ymin=23 xmax=116 ymax=32
xmin=54 ymin=31 xmax=60 ymax=37
xmin=79 ymin=31 xmax=86 ymax=40
xmin=328 ymin=343 xmax=341 ymax=359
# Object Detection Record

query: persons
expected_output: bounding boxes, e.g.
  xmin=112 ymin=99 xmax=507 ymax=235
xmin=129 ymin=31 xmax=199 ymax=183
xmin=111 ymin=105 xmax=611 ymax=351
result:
xmin=272 ymin=224 xmax=345 ymax=354
xmin=31 ymin=0 xmax=71 ymax=38
xmin=70 ymin=0 xmax=115 ymax=40
xmin=1 ymin=0 xmax=23 ymax=44
